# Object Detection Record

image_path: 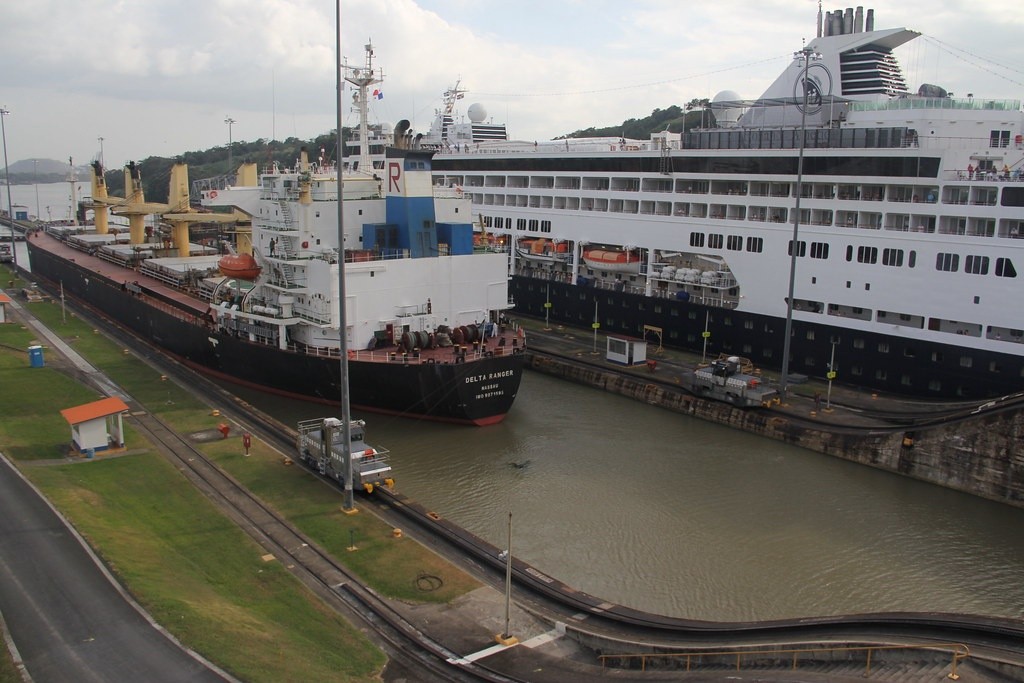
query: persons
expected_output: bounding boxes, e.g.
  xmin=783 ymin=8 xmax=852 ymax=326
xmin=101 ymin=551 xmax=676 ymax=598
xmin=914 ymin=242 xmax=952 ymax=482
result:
xmin=1010 ymin=226 xmax=1018 ymax=238
xmin=491 ymin=321 xmax=498 ymax=337
xmin=533 ymin=141 xmax=538 ymax=152
xmin=677 ymin=187 xmax=936 ymax=232
xmin=619 ymin=139 xmax=628 ymax=152
xmin=968 ymin=164 xmax=1022 ymax=182
xmin=565 ymin=140 xmax=570 ymax=153
xmin=270 ymin=238 xmax=276 ymax=256
xmin=456 ymin=142 xmax=480 ymax=152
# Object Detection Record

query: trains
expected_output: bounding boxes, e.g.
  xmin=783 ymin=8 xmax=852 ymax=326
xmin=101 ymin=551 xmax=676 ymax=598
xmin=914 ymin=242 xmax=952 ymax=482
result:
xmin=691 ymin=355 xmax=782 ymax=409
xmin=296 ymin=416 xmax=396 ymax=495
xmin=0 ymin=244 xmax=15 ymax=263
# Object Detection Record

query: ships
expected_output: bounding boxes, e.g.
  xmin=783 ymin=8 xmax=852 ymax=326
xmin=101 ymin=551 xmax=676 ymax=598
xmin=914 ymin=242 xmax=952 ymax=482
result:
xmin=22 ymin=41 xmax=531 ymax=430
xmin=428 ymin=1 xmax=1024 ymax=409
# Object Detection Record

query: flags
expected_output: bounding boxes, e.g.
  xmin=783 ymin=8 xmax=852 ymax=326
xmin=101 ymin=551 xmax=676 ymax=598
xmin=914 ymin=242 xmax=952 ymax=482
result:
xmin=372 ymin=89 xmax=383 ymax=99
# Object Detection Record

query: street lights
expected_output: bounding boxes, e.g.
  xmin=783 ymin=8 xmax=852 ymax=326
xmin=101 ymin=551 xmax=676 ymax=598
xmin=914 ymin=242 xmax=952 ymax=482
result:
xmin=224 ymin=118 xmax=237 ymax=171
xmin=0 ymin=107 xmax=21 ymax=281
xmin=777 ymin=47 xmax=823 ymax=406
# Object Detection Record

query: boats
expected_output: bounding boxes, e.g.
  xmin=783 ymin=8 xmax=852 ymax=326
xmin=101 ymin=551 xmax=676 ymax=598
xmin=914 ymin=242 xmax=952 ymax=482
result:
xmin=515 ymin=237 xmax=569 ymax=264
xmin=581 ymin=249 xmax=641 ymax=276
xmin=217 ymin=252 xmax=261 ymax=278
